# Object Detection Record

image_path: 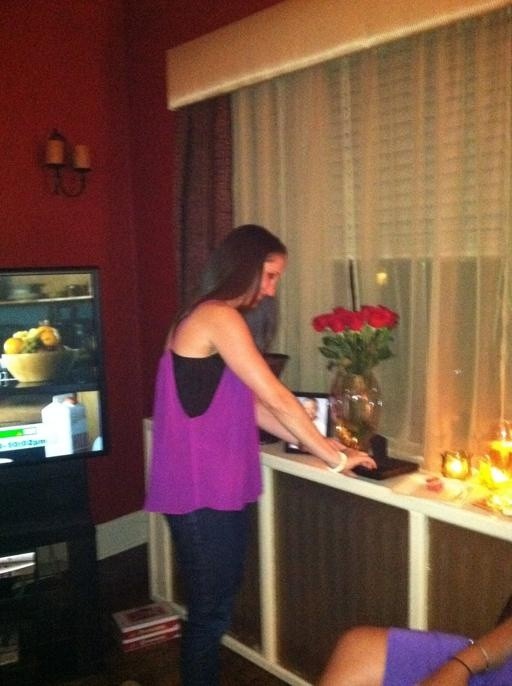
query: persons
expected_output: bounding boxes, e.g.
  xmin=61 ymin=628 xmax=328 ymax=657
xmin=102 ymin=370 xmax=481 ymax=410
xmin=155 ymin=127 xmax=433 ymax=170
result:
xmin=301 ymin=397 xmax=325 ymax=434
xmin=146 ymin=224 xmax=378 ymax=686
xmin=315 ymin=593 xmax=511 ymax=686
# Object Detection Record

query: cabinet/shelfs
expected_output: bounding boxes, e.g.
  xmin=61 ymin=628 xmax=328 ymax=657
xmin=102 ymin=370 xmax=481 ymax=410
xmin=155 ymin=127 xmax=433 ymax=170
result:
xmin=134 ymin=395 xmax=512 ymax=686
xmin=0 ymin=450 xmax=111 ymax=686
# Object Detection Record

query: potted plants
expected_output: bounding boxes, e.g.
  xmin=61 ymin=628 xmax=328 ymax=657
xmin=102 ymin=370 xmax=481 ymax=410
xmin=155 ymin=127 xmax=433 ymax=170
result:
xmin=233 ymin=291 xmax=296 ymax=446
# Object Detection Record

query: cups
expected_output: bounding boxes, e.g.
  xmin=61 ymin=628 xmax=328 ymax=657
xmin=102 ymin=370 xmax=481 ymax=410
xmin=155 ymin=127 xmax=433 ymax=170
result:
xmin=438 ymin=450 xmax=472 ymax=481
xmin=474 ymin=419 xmax=512 ymax=495
xmin=65 ymin=284 xmax=80 ymax=296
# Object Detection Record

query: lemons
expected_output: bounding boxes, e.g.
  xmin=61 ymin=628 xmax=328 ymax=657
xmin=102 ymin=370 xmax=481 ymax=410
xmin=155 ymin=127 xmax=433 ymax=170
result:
xmin=4 ymin=326 xmax=60 ymax=354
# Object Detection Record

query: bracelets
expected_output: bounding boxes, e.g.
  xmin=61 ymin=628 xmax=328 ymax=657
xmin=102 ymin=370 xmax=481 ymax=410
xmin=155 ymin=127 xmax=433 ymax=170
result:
xmin=327 ymin=451 xmax=349 ymax=474
xmin=469 ymin=639 xmax=494 ymax=674
xmin=453 ymin=654 xmax=475 ymax=680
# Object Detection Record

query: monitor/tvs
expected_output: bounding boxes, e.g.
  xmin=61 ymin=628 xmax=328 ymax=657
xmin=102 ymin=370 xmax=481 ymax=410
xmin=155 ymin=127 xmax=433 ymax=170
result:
xmin=0 ymin=267 xmax=108 ymax=465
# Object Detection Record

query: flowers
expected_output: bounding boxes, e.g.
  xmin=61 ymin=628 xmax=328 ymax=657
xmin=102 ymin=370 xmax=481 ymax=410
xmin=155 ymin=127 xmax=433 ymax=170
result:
xmin=306 ymin=299 xmax=403 ymax=441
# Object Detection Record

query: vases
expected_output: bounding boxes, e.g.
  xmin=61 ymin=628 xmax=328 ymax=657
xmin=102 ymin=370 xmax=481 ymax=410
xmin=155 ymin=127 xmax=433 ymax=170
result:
xmin=325 ymin=371 xmax=388 ymax=455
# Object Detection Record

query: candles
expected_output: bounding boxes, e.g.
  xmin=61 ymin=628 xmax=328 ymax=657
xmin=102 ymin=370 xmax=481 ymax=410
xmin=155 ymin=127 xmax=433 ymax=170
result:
xmin=42 ymin=134 xmax=65 ymax=165
xmin=71 ymin=143 xmax=93 ymax=170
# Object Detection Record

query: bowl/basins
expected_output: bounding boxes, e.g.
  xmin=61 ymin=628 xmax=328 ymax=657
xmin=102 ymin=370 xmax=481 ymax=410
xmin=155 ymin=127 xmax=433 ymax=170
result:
xmin=1 ymin=348 xmax=80 ymax=384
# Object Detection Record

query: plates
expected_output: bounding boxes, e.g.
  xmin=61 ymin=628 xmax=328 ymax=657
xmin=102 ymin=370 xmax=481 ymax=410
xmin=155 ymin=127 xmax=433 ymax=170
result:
xmin=6 ymin=282 xmax=47 ymax=301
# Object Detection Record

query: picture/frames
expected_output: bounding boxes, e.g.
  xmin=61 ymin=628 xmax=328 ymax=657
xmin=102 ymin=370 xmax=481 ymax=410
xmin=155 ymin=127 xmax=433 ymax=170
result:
xmin=282 ymin=389 xmax=328 ymax=451
